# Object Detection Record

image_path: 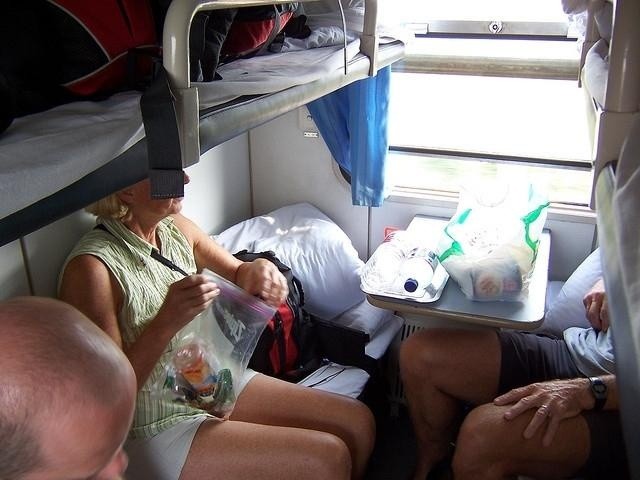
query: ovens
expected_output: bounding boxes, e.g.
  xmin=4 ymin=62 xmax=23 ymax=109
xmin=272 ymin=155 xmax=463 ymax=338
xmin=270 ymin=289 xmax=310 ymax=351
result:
xmin=0 ymin=33 xmax=406 ymax=246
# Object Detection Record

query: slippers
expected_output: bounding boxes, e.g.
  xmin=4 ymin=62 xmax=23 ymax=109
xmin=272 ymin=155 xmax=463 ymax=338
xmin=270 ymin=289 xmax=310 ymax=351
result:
xmin=212 ymin=249 xmax=324 ymax=384
xmin=1 ymin=0 xmax=299 ymax=132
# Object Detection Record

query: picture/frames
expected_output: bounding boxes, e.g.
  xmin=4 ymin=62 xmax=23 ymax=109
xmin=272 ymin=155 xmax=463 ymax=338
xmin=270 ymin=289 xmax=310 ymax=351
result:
xmin=366 ymin=216 xmax=553 ymax=330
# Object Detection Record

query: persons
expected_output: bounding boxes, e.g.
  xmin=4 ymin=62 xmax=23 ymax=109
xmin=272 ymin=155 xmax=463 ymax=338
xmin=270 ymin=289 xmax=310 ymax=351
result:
xmin=398 ymin=277 xmax=629 ymax=480
xmin=1 ymin=295 xmax=137 ymax=480
xmin=57 ymin=169 xmax=377 ymax=480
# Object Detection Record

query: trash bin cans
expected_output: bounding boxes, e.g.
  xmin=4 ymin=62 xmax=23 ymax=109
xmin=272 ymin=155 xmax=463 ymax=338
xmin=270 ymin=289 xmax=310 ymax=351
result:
xmin=588 ymin=375 xmax=608 ymax=412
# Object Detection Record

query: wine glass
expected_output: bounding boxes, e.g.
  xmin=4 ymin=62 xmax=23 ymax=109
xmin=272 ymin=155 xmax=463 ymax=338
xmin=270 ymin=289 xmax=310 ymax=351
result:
xmin=541 ymin=405 xmax=552 ymax=414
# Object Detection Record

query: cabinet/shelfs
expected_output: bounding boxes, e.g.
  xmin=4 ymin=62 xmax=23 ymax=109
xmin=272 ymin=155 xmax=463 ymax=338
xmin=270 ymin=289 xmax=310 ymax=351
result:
xmin=173 ymin=344 xmax=218 ymax=396
xmin=360 ymin=231 xmax=439 ymax=295
